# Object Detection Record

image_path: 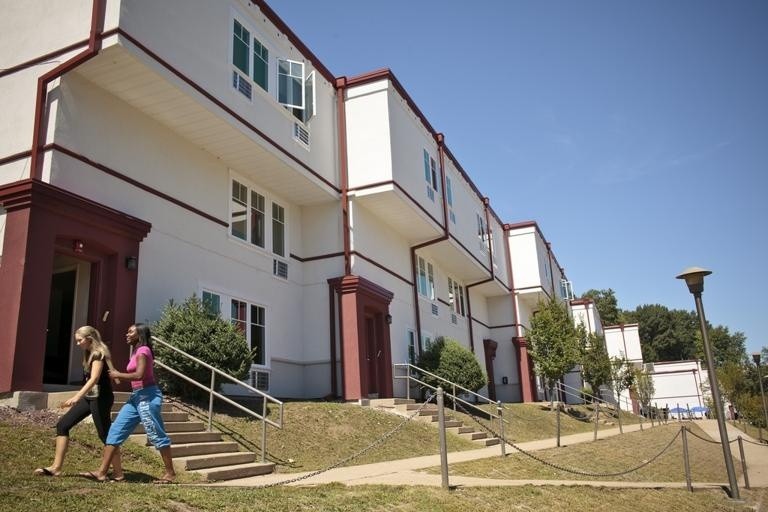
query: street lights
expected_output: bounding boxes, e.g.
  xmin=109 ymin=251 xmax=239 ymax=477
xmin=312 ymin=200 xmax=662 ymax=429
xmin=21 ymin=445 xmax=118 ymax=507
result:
xmin=752 ymin=352 xmax=767 ymax=426
xmin=675 ymin=268 xmax=739 ymax=501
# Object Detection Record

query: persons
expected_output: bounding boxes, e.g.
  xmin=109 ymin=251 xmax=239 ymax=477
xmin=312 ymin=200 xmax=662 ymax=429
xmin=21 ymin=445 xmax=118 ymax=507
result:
xmin=35 ymin=326 xmax=127 ymax=483
xmin=79 ymin=324 xmax=176 ymax=484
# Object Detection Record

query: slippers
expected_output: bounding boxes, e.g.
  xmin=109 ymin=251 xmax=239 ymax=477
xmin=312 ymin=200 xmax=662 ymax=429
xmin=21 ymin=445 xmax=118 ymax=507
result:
xmin=36 ymin=468 xmax=59 ymax=476
xmin=80 ymin=471 xmax=125 ymax=482
xmin=153 ymin=479 xmax=177 ymax=483
